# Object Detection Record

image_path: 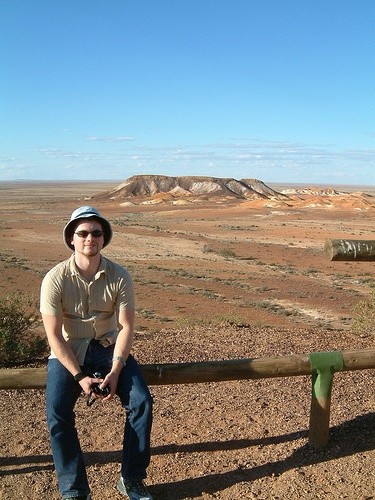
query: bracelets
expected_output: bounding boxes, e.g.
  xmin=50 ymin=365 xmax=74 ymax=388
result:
xmin=74 ymin=372 xmax=85 ymax=383
xmin=111 ymin=356 xmax=127 ymax=367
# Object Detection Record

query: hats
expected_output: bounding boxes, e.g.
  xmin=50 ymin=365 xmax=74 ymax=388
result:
xmin=63 ymin=206 xmax=113 ymax=253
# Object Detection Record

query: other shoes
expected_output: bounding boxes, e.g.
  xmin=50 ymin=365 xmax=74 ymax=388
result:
xmin=117 ymin=475 xmax=153 ymax=500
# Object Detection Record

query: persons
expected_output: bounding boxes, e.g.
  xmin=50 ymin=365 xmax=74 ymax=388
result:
xmin=40 ymin=206 xmax=152 ymax=500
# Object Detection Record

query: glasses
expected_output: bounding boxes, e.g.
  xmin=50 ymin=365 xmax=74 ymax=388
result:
xmin=70 ymin=228 xmax=105 ymax=239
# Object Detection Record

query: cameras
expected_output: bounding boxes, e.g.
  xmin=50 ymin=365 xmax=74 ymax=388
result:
xmin=92 ymin=372 xmax=110 ymax=397
